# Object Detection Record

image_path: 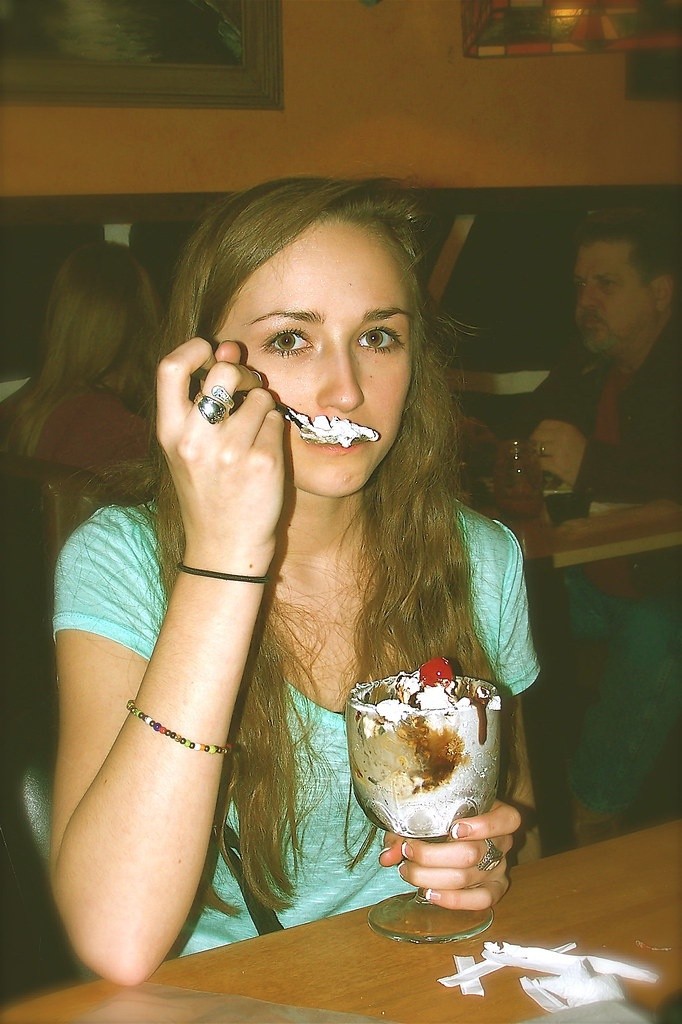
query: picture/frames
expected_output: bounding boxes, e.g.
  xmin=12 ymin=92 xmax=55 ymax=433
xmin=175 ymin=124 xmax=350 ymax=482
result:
xmin=624 ymin=48 xmax=682 ymax=103
xmin=0 ymin=2 xmax=285 ymax=112
xmin=458 ymin=0 xmax=682 ymax=57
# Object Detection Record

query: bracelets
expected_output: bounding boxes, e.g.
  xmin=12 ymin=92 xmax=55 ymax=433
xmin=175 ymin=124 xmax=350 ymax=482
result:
xmin=128 ymin=700 xmax=234 ymax=756
xmin=176 ymin=559 xmax=270 ymax=584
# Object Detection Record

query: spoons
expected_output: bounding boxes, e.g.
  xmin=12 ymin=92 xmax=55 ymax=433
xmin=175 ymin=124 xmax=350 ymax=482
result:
xmin=196 ymin=368 xmax=381 ymax=442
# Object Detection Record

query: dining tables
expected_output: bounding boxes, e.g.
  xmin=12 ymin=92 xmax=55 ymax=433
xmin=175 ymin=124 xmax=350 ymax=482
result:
xmin=472 ymin=491 xmax=682 ymax=567
xmin=1 ymin=814 xmax=682 ymax=1024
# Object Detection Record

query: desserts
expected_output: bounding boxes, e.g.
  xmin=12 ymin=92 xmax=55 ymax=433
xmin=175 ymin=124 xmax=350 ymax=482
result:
xmin=358 ymin=659 xmax=500 ymax=838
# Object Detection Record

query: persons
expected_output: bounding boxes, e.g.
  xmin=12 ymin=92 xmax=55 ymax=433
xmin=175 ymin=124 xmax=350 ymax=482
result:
xmin=1 ymin=244 xmax=162 ymax=504
xmin=51 ymin=176 xmax=540 ymax=985
xmin=457 ymin=204 xmax=681 ymax=848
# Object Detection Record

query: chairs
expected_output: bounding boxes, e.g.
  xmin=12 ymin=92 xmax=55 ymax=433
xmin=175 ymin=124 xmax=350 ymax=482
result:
xmin=1 ymin=455 xmax=52 ymax=739
xmin=1 ymin=471 xmax=542 ymax=992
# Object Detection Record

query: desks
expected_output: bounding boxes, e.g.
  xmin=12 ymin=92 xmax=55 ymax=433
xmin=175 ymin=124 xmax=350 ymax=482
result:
xmin=442 ymin=366 xmax=550 ymax=398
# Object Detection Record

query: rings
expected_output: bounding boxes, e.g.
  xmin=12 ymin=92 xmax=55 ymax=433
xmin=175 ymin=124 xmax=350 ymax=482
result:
xmin=193 ymin=385 xmax=235 ymax=425
xmin=477 ymin=837 xmax=502 ymax=872
xmin=541 ymin=443 xmax=544 ymax=457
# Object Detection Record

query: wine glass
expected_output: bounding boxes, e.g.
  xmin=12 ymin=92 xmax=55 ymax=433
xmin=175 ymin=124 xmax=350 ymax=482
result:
xmin=344 ymin=674 xmax=500 ymax=943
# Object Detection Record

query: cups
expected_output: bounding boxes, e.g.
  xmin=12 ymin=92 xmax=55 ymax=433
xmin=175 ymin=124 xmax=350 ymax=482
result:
xmin=494 ymin=440 xmax=542 ymax=519
xmin=543 ymin=494 xmax=591 ymax=525
